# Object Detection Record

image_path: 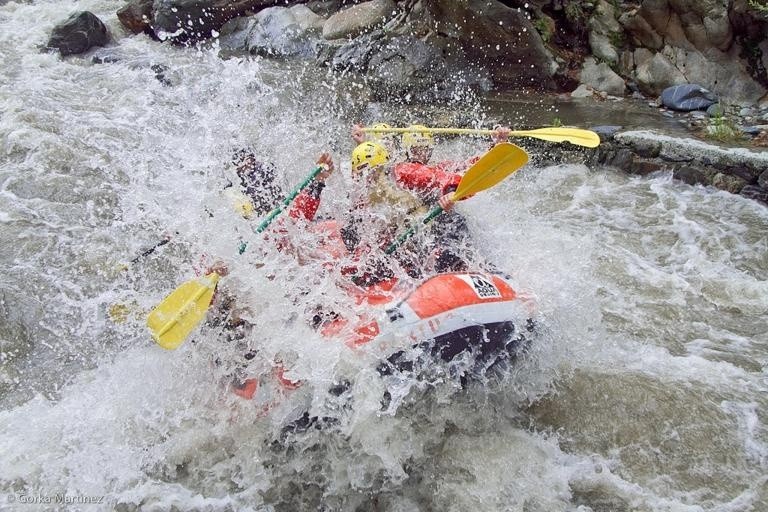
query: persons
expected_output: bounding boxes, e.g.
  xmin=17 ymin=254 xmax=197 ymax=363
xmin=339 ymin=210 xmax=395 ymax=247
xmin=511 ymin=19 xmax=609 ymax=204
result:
xmin=221 ymin=146 xmax=291 ymax=228
xmin=289 ymin=124 xmax=511 ymax=290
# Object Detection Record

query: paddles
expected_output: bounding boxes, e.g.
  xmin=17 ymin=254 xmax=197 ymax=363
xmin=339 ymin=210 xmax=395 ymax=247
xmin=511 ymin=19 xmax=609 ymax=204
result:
xmin=384 ymin=142 xmax=528 ymax=256
xmin=357 ymin=128 xmax=600 ymax=148
xmin=144 ymin=165 xmax=321 ymax=351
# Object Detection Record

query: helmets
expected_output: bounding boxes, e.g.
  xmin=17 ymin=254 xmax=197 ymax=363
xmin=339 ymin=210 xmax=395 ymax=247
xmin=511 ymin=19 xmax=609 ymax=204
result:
xmin=351 ymin=124 xmax=434 ymax=171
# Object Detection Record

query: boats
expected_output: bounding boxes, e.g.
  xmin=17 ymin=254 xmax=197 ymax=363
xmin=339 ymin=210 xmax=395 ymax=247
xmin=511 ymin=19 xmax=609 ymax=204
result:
xmin=227 ymin=222 xmax=526 ymax=413
